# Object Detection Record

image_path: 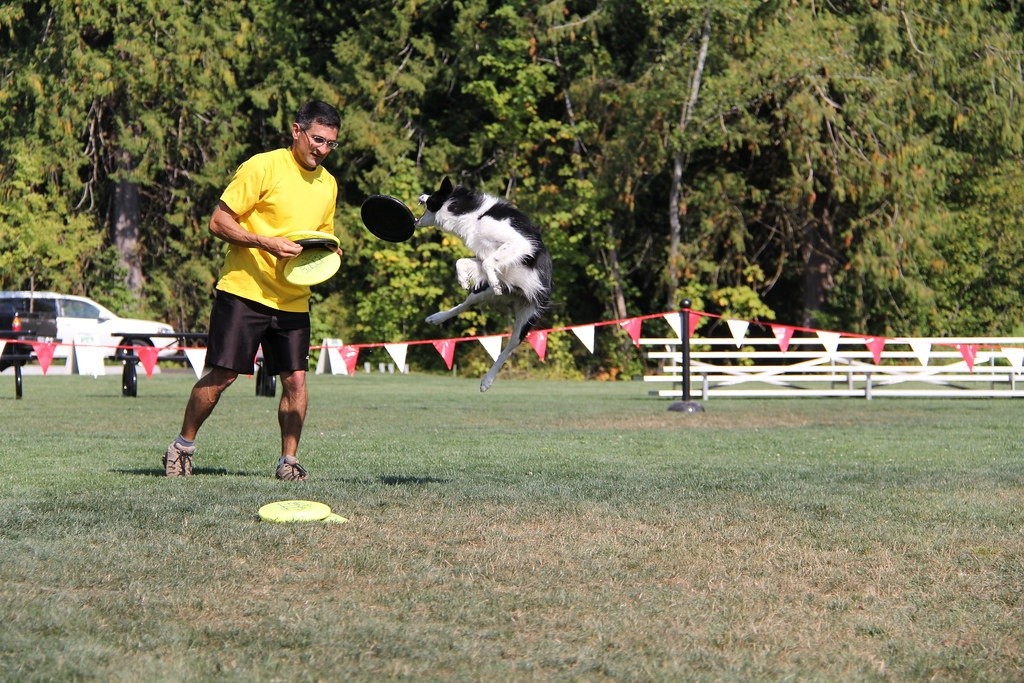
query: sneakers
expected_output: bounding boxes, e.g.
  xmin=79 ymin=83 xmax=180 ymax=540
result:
xmin=275 ymin=455 xmax=308 ymax=481
xmin=161 ymin=441 xmax=196 ymax=477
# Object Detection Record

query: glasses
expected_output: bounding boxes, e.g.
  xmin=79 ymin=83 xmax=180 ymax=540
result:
xmin=301 ymin=125 xmax=338 ymax=150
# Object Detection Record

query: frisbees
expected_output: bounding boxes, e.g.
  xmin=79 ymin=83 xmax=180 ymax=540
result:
xmin=282 ymin=232 xmax=341 ymax=249
xmin=360 ymin=194 xmax=416 ymax=243
xmin=256 ymin=499 xmax=333 ymax=523
xmin=283 ymin=249 xmax=341 ymax=287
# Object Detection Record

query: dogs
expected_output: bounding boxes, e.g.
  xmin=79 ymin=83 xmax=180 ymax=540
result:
xmin=414 ymin=175 xmax=553 ymax=394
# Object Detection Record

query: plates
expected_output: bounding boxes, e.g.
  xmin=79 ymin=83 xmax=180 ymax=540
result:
xmin=284 ymin=246 xmax=341 ymax=287
xmin=361 ymin=195 xmax=415 ymax=243
xmin=258 ymin=500 xmax=330 ymax=523
xmin=284 ymin=231 xmax=341 ymax=249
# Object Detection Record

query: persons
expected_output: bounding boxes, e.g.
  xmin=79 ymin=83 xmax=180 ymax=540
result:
xmin=164 ymin=101 xmax=343 ymax=483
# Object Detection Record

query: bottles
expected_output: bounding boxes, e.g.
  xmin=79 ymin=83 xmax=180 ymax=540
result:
xmin=12 ymin=312 xmax=21 ymax=331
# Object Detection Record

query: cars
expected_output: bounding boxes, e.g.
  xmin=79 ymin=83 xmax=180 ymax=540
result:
xmin=4 ymin=290 xmax=179 ymax=369
